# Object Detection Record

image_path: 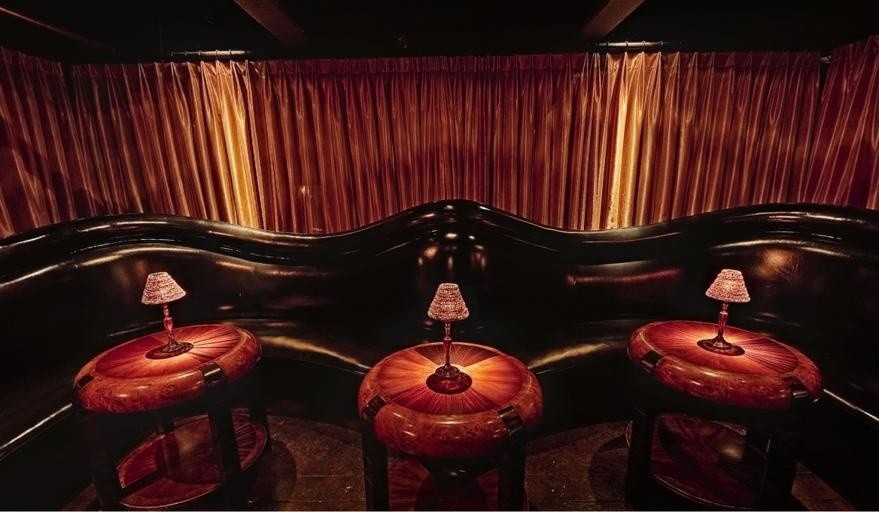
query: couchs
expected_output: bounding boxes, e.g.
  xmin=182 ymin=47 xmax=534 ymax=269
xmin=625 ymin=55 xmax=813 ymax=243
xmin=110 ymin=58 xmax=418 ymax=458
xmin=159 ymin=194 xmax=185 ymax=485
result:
xmin=0 ymin=199 xmax=879 ymax=511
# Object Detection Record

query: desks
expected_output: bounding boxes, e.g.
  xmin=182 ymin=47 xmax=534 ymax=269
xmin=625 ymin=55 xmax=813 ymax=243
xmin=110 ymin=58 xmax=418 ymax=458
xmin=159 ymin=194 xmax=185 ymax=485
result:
xmin=624 ymin=322 xmax=823 ymax=511
xmin=358 ymin=343 xmax=543 ymax=511
xmin=73 ymin=324 xmax=270 ymax=511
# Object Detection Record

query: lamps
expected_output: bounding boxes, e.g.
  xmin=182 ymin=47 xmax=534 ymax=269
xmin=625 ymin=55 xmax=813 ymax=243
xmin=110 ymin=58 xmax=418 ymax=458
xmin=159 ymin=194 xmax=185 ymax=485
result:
xmin=141 ymin=271 xmax=186 ymax=352
xmin=427 ymin=283 xmax=469 ymax=378
xmin=705 ymin=269 xmax=750 ymax=348
